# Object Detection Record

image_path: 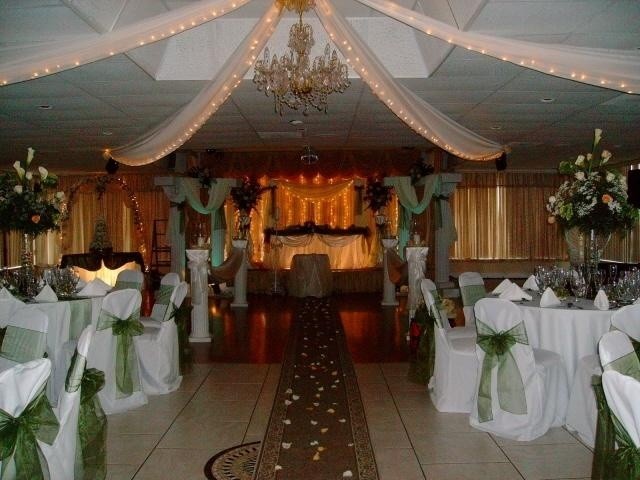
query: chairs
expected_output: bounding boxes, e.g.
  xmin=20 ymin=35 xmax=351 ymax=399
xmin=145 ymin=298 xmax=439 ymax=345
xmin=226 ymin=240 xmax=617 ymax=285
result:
xmin=50 ymin=324 xmax=108 ymax=480
xmin=2 ymin=359 xmax=61 ymax=480
xmin=459 ymin=271 xmax=487 ymax=327
xmin=139 ymin=281 xmax=188 ymax=397
xmin=474 ymin=300 xmax=556 ymax=444
xmin=595 ymin=331 xmax=640 ymax=375
xmin=2 ymin=308 xmax=49 ymax=368
xmin=596 ymin=371 xmax=639 ymax=479
xmin=56 ymin=289 xmax=148 ymax=416
xmin=116 ymin=268 xmax=145 ymax=290
xmin=420 ymin=279 xmax=473 ymax=416
xmin=610 ymin=306 xmax=640 ymax=335
xmin=140 ymin=271 xmax=181 ymax=328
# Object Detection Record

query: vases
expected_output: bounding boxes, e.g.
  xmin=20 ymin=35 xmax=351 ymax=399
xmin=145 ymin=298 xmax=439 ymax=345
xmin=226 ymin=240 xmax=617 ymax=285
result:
xmin=577 ymin=229 xmax=603 ymax=299
xmin=198 ymin=187 xmax=212 ymax=207
xmin=235 ymin=216 xmax=251 ymax=235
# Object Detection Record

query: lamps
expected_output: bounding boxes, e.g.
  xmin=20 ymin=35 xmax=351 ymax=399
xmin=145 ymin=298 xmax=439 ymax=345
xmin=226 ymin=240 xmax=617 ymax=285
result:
xmin=252 ymin=0 xmax=353 ymax=120
xmin=297 ymin=143 xmax=322 ymax=164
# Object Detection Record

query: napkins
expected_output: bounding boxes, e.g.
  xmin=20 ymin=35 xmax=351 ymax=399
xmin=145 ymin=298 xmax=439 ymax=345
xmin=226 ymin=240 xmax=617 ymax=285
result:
xmin=1 ymin=287 xmax=18 ymax=303
xmin=77 ymin=277 xmax=108 ymax=297
xmin=36 ymin=285 xmax=59 ymax=303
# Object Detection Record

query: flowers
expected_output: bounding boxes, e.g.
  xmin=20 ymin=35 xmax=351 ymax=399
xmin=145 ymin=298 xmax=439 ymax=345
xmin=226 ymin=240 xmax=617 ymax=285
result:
xmin=198 ymin=167 xmax=217 ymax=188
xmin=17 ymin=238 xmax=41 ymax=298
xmin=0 ymin=146 xmax=68 ymax=237
xmin=365 ymin=180 xmax=397 ymax=212
xmin=232 ymin=172 xmax=261 ymax=213
xmin=549 ymin=121 xmax=639 ymax=227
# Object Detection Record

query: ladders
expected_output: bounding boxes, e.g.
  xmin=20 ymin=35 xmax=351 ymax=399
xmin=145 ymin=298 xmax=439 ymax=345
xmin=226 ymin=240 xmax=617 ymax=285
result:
xmin=148 ymin=218 xmax=172 ymax=276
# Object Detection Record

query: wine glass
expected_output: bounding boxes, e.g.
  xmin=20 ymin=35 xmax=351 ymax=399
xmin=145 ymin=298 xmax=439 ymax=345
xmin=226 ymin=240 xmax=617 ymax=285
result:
xmin=534 ymin=264 xmax=640 ymax=307
xmin=0 ymin=266 xmax=83 ymax=301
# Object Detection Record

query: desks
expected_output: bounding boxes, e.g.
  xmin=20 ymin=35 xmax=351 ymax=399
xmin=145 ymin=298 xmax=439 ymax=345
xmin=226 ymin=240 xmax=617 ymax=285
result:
xmin=61 ymin=253 xmax=148 ymax=285
